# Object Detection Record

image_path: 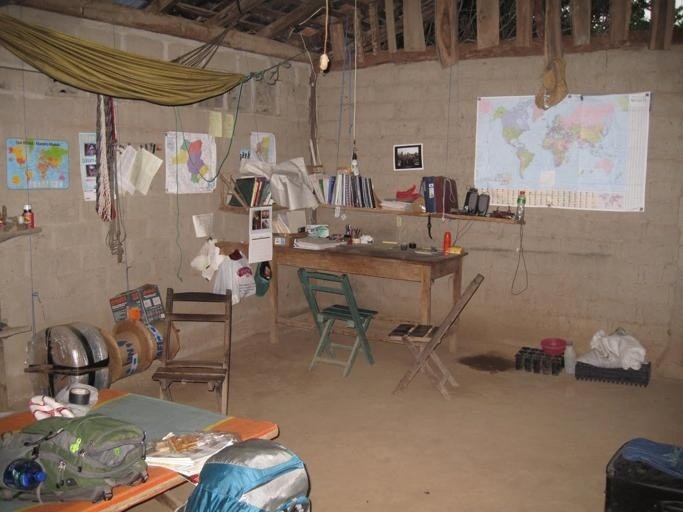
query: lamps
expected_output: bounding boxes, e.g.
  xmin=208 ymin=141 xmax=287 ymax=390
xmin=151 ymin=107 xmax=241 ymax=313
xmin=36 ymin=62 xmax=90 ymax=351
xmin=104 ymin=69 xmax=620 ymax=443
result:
xmin=351 ymin=0 xmax=360 ymax=177
xmin=319 ymin=0 xmax=329 ymax=70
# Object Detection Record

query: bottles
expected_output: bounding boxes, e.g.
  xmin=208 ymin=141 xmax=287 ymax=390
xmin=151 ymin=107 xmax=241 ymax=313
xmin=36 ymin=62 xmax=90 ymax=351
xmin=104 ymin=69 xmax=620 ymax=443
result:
xmin=514 ymin=191 xmax=526 ymax=221
xmin=462 ymin=186 xmax=490 ymax=216
xmin=443 ymin=232 xmax=451 ymax=252
xmin=3 ymin=459 xmax=47 ymax=491
xmin=562 ymin=343 xmax=576 ymax=374
xmin=21 ymin=204 xmax=35 ymax=230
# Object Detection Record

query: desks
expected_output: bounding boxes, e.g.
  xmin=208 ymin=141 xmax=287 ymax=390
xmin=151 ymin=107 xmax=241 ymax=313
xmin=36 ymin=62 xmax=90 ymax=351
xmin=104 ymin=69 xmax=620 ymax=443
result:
xmin=0 ymin=388 xmax=279 ymax=512
xmin=269 ymin=242 xmax=468 ymax=353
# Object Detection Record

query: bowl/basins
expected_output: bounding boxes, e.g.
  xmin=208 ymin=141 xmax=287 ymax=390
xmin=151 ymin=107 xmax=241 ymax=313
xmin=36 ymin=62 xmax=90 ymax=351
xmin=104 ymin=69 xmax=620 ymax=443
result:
xmin=540 ymin=337 xmax=565 ymax=355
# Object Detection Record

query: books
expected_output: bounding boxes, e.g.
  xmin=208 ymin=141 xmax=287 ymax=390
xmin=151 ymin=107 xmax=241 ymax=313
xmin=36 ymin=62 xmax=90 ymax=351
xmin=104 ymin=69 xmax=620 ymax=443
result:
xmin=227 ymin=175 xmax=273 ymax=208
xmin=294 ymin=237 xmax=347 ymax=251
xmin=308 ymin=172 xmax=380 ymax=209
xmin=144 ymin=432 xmax=235 ymax=486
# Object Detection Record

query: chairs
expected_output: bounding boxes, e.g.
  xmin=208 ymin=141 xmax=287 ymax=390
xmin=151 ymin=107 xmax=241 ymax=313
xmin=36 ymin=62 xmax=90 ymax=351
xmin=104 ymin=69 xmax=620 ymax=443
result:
xmin=387 ymin=273 xmax=485 ymax=399
xmin=295 ymin=268 xmax=379 ymax=379
xmin=151 ymin=286 xmax=234 ymax=416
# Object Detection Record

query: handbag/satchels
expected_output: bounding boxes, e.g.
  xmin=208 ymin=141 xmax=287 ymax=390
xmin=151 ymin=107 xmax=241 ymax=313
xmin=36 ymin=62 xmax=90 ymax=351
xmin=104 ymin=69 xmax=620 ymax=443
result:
xmin=535 ymin=53 xmax=568 ymax=111
xmin=184 ymin=439 xmax=311 ymax=512
xmin=0 ymin=414 xmax=149 ymax=504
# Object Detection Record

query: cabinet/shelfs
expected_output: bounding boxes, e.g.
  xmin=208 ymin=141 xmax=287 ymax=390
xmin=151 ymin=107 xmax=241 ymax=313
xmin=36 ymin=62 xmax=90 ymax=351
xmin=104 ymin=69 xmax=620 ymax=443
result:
xmin=0 ymin=227 xmax=44 ymax=340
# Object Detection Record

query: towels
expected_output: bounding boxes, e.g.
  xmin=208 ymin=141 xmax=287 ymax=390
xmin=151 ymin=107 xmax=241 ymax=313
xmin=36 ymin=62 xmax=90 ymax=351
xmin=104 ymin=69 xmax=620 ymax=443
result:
xmin=27 ymin=381 xmax=98 ymax=422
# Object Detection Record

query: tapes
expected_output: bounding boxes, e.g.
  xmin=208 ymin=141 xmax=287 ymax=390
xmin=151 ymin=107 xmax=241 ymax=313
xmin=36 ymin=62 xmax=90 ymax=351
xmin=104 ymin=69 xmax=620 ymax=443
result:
xmin=69 ymin=388 xmax=90 ymax=405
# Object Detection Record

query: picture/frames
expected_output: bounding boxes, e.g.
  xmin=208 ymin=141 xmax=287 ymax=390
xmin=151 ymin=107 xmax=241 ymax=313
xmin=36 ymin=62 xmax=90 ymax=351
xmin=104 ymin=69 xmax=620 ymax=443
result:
xmin=392 ymin=143 xmax=424 ymax=172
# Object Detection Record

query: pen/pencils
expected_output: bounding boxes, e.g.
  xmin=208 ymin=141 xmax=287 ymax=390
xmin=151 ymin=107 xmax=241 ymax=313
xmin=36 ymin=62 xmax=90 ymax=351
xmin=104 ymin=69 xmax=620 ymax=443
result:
xmin=345 ymin=223 xmax=360 ymax=235
